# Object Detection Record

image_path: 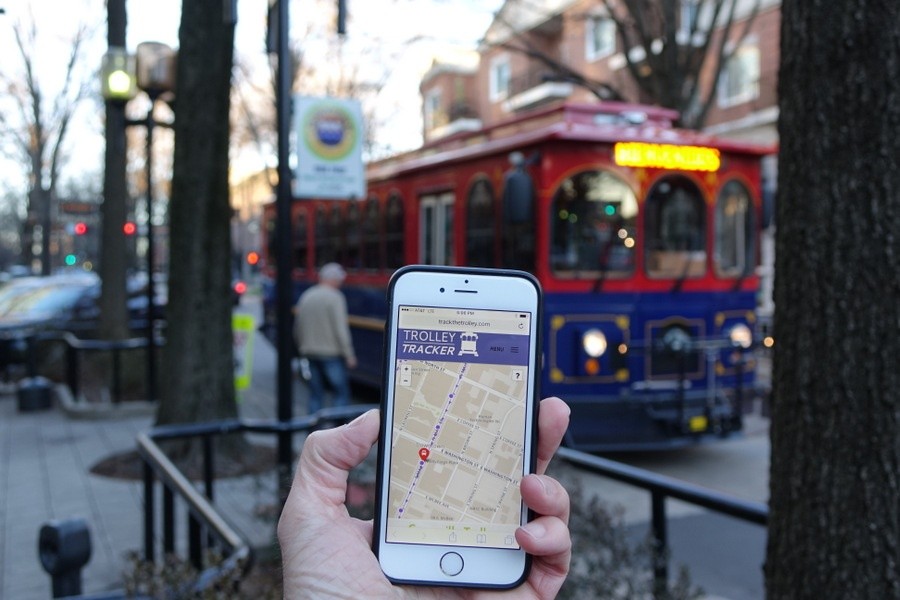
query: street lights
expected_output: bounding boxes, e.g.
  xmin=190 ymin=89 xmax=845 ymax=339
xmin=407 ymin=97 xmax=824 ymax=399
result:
xmin=101 ymin=42 xmax=176 ymax=401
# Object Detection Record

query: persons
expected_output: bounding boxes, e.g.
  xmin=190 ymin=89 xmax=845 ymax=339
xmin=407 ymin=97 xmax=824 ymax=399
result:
xmin=290 ymin=263 xmax=358 ymax=430
xmin=276 ymin=395 xmax=571 ymax=600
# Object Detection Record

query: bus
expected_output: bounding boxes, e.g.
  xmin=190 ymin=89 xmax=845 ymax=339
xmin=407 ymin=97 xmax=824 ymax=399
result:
xmin=260 ymin=100 xmax=778 ymax=466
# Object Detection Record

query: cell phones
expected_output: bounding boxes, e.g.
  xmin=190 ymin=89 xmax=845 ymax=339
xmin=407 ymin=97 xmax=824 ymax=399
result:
xmin=367 ymin=265 xmax=544 ymax=589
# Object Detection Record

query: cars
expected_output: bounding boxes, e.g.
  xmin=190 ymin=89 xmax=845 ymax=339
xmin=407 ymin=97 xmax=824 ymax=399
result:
xmin=0 ymin=270 xmax=168 ymax=396
